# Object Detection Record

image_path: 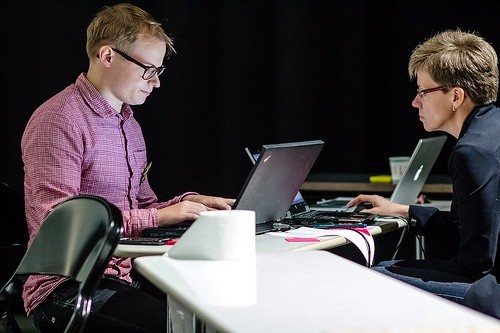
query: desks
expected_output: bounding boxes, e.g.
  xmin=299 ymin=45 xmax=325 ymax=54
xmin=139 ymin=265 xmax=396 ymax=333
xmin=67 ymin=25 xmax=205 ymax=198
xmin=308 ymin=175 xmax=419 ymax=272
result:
xmin=300 ymin=183 xmax=453 ymax=193
xmin=114 ymin=200 xmax=452 ymax=258
xmin=135 ymin=251 xmax=500 ymax=333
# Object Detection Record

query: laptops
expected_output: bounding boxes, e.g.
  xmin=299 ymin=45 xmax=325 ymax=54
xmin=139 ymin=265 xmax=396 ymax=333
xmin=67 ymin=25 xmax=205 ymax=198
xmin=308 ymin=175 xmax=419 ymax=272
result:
xmin=390 ymin=136 xmax=448 ymax=205
xmin=245 ymin=146 xmax=376 ymax=224
xmin=141 ymin=140 xmax=325 ymax=238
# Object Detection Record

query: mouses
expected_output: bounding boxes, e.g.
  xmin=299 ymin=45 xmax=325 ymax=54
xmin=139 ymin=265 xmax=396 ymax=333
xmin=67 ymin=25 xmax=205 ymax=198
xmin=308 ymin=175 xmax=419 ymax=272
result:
xmin=346 ymin=203 xmax=374 ymax=213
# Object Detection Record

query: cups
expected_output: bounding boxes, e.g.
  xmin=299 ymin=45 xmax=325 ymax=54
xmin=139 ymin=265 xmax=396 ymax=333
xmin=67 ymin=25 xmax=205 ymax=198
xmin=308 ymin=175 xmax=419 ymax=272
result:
xmin=389 ymin=157 xmax=411 ymax=184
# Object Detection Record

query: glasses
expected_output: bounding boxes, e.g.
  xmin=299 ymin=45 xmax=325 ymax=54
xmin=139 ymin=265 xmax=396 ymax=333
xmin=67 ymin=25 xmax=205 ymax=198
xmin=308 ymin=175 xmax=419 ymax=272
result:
xmin=415 ymin=85 xmax=457 ymax=99
xmin=96 ymin=47 xmax=166 ymax=80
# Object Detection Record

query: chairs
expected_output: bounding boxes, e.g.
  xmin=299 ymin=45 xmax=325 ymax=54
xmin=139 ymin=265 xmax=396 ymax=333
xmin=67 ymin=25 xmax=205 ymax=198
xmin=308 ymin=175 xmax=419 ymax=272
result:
xmin=0 ymin=195 xmax=123 ymax=333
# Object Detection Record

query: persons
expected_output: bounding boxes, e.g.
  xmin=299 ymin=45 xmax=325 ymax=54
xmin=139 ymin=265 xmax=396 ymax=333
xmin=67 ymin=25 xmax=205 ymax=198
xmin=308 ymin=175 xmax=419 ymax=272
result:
xmin=21 ymin=3 xmax=238 ymax=333
xmin=347 ymin=26 xmax=500 ymax=305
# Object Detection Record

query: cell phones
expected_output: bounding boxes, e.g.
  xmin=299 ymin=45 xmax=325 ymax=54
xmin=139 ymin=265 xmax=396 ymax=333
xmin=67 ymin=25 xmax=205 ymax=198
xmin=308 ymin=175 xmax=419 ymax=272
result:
xmin=119 ymin=238 xmax=171 ymax=245
